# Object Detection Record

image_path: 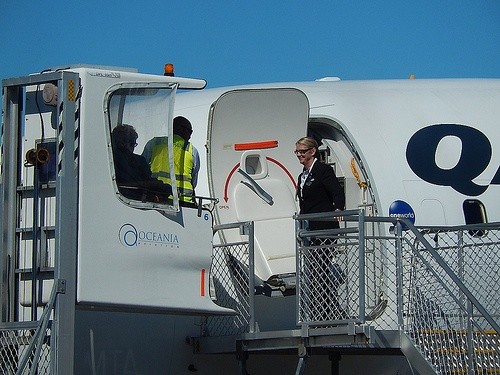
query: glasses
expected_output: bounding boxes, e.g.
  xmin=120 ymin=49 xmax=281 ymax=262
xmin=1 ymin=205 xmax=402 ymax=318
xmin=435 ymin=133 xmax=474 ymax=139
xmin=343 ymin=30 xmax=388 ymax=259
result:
xmin=131 ymin=143 xmax=138 ymax=147
xmin=294 ymin=148 xmax=313 ymax=155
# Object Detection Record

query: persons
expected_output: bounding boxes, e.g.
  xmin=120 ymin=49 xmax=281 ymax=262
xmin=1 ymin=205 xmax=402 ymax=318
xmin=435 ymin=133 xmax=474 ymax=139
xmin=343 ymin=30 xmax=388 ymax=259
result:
xmin=293 ymin=135 xmax=345 ymax=329
xmin=111 ymin=122 xmax=173 ymax=206
xmin=140 ymin=116 xmax=200 ymax=205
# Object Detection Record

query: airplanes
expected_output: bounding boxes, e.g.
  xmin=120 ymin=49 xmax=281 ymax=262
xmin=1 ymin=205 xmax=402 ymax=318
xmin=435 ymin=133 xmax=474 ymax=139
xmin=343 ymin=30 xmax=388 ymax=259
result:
xmin=172 ymin=77 xmax=500 ymax=330
